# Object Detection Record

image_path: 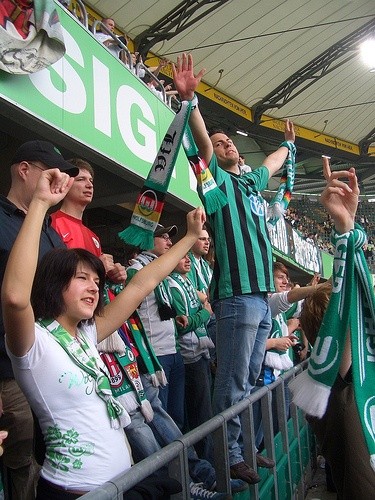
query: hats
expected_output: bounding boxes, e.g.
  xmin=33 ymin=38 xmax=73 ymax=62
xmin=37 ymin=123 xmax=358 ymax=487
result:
xmin=154 ymin=224 xmax=178 ymax=236
xmin=10 ymin=140 xmax=79 ymax=176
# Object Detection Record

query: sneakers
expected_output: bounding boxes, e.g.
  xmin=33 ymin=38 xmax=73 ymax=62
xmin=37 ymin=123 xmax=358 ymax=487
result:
xmin=189 ymin=485 xmax=231 ymax=500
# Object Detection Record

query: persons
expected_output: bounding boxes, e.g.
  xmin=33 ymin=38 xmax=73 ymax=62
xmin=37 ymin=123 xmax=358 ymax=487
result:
xmin=167 ymin=252 xmax=216 ymax=461
xmin=0 ymin=141 xmax=80 ymax=500
xmin=92 ymin=18 xmax=179 ymax=109
xmin=122 ymin=223 xmax=188 ymax=433
xmin=252 ymin=261 xmax=331 ymax=452
xmin=2 ymin=168 xmax=206 ymax=500
xmin=299 ymin=154 xmax=375 ymax=500
xmin=49 ymin=157 xmax=249 ymax=500
xmin=259 ymin=192 xmax=375 ymax=275
xmin=170 ymin=52 xmax=294 ymax=484
xmin=185 ymin=224 xmax=214 ymax=305
xmin=237 ymin=155 xmax=252 ymax=176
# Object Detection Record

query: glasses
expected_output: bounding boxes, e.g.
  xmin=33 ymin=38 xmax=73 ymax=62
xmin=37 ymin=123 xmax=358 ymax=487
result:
xmin=154 ymin=236 xmax=172 ymax=241
xmin=198 ymin=238 xmax=212 ymax=242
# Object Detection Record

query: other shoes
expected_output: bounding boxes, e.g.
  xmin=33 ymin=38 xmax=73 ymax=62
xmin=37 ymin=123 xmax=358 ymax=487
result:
xmin=230 ymin=462 xmax=260 ymax=484
xmin=211 ymin=478 xmax=248 ymax=491
xmin=254 ymin=453 xmax=275 ymax=468
